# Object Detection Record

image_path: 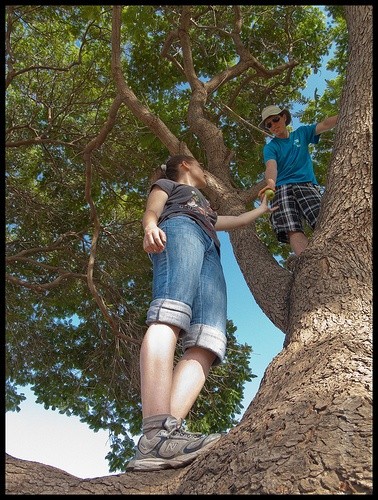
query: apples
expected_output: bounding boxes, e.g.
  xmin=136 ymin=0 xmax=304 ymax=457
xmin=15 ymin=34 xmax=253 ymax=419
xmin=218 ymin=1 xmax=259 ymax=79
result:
xmin=264 ymin=189 xmax=275 ymax=200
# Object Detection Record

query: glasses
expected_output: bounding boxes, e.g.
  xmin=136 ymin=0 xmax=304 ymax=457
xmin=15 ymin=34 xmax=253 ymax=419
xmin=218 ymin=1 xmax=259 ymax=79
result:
xmin=265 ymin=115 xmax=282 ymax=128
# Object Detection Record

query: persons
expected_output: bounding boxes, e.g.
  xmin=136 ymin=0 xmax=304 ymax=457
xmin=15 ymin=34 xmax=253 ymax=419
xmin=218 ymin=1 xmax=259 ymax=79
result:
xmin=258 ymin=106 xmax=338 ymax=255
xmin=126 ymin=156 xmax=278 ymax=469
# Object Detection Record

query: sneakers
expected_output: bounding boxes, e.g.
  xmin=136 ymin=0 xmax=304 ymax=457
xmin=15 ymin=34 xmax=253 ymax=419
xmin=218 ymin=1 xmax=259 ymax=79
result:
xmin=126 ymin=458 xmax=134 ymax=470
xmin=134 ymin=417 xmax=222 ymax=470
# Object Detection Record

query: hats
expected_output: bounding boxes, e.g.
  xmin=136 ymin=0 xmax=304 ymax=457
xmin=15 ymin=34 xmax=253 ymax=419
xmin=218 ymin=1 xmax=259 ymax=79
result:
xmin=258 ymin=105 xmax=292 ymax=132
